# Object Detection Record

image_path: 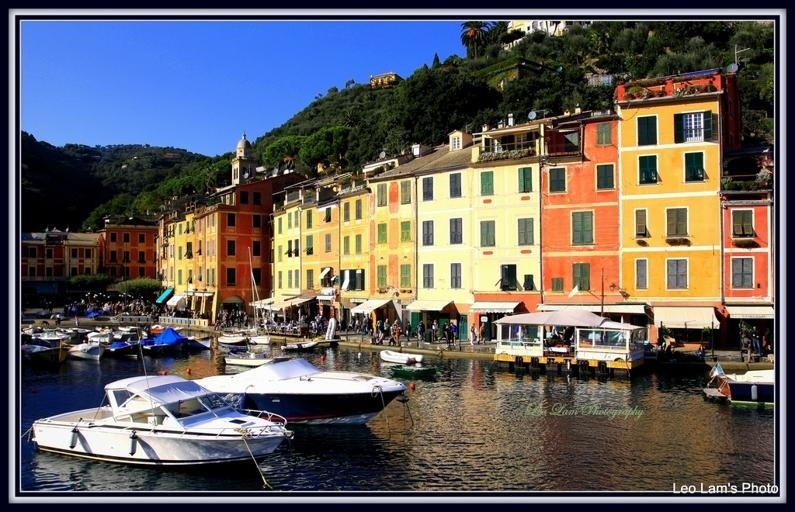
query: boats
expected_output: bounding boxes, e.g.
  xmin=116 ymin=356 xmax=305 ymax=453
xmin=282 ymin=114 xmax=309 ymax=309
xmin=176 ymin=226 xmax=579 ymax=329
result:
xmin=181 ymin=355 xmax=415 ymax=432
xmin=29 ymin=370 xmax=303 ymax=468
xmin=703 ymin=367 xmax=775 ymax=413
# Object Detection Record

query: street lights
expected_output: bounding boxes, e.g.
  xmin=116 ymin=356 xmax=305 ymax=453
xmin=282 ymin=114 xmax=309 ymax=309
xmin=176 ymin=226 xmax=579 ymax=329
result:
xmin=83 ymin=289 xmax=133 ymax=305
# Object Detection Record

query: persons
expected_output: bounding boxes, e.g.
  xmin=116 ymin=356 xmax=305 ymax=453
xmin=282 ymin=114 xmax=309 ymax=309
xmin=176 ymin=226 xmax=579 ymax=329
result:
xmin=513 ymin=322 xmax=773 ymax=365
